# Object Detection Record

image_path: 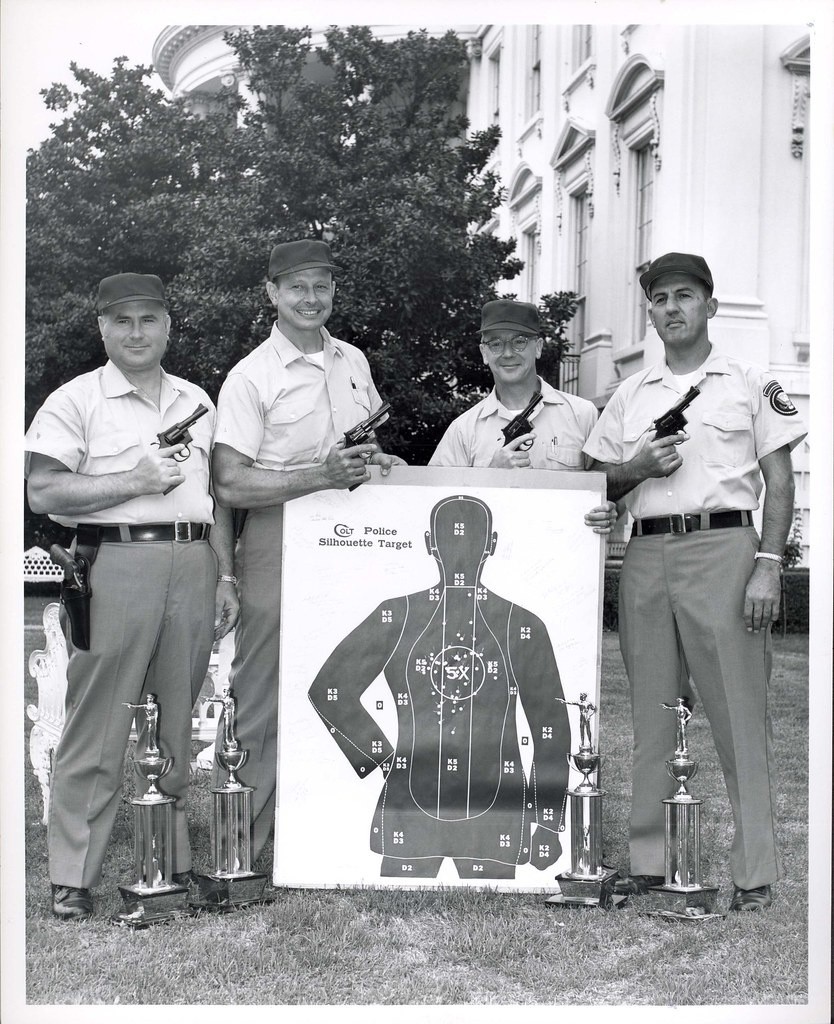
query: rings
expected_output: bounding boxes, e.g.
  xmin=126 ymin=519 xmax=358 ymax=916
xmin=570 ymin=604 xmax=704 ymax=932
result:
xmin=605 ymin=520 xmax=611 ymax=528
xmin=230 ymin=627 xmax=235 ymax=632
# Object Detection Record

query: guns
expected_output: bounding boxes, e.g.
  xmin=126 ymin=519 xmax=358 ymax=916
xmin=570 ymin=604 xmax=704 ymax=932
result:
xmin=335 ymin=404 xmax=392 ymax=491
xmin=652 ymin=386 xmax=703 ymax=479
xmin=147 ymin=403 xmax=208 ymax=495
xmin=50 ymin=542 xmax=88 ymax=593
xmin=495 ymin=391 xmax=541 ymax=454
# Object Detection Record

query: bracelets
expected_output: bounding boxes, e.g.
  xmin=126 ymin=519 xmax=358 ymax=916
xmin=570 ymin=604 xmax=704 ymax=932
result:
xmin=217 ymin=575 xmax=238 ymax=586
xmin=754 ymin=552 xmax=781 ymax=564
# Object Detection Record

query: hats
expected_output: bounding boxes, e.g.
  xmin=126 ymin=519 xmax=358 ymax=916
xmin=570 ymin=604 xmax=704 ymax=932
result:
xmin=268 ymin=239 xmax=343 ymax=282
xmin=97 ymin=272 xmax=170 ymax=314
xmin=639 ymin=253 xmax=713 ymax=301
xmin=475 ymin=299 xmax=540 ymax=337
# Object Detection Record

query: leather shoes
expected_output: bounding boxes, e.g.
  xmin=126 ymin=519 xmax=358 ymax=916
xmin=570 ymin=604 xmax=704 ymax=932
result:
xmin=52 ymin=883 xmax=93 ymax=922
xmin=728 ymin=883 xmax=772 ymax=911
xmin=143 ymin=871 xmax=208 ymax=908
xmin=614 ymin=873 xmax=673 ymax=895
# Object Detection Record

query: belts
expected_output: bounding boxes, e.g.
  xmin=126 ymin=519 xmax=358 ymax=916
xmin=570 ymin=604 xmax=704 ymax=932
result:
xmin=630 ymin=509 xmax=754 ymax=538
xmin=76 ymin=522 xmax=211 ymax=542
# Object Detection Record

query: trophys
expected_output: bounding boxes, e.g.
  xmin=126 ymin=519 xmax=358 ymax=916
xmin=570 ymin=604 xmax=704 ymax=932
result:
xmin=186 ymin=689 xmax=275 ymax=913
xmin=109 ymin=693 xmax=200 ymax=929
xmin=556 ymin=693 xmax=628 ymax=911
xmin=637 ymin=697 xmax=726 ymax=924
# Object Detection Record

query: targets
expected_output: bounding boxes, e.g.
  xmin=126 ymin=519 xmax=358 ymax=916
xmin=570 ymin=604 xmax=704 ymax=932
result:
xmin=272 ymin=459 xmax=608 ymax=895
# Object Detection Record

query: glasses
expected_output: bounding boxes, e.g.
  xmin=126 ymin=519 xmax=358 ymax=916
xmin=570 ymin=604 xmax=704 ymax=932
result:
xmin=482 ymin=334 xmax=538 ymax=354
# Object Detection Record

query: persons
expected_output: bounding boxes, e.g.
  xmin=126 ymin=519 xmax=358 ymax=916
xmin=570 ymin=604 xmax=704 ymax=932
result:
xmin=23 ymin=270 xmax=241 ymax=920
xmin=210 ymin=236 xmax=408 ymax=874
xmin=583 ymin=252 xmax=810 ymax=911
xmin=428 ymin=299 xmax=626 ymax=534
